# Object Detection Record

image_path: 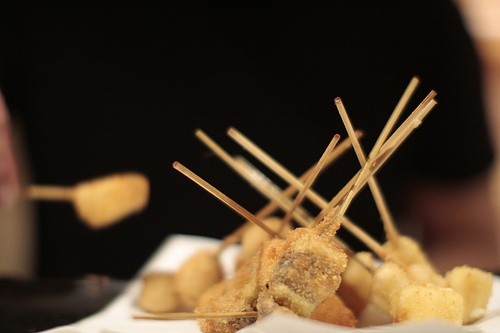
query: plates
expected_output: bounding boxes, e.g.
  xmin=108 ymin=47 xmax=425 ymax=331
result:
xmin=41 ymin=233 xmax=500 ymax=333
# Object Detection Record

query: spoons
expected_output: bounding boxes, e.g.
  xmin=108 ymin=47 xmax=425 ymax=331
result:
xmin=19 ymin=171 xmax=150 ymax=228
